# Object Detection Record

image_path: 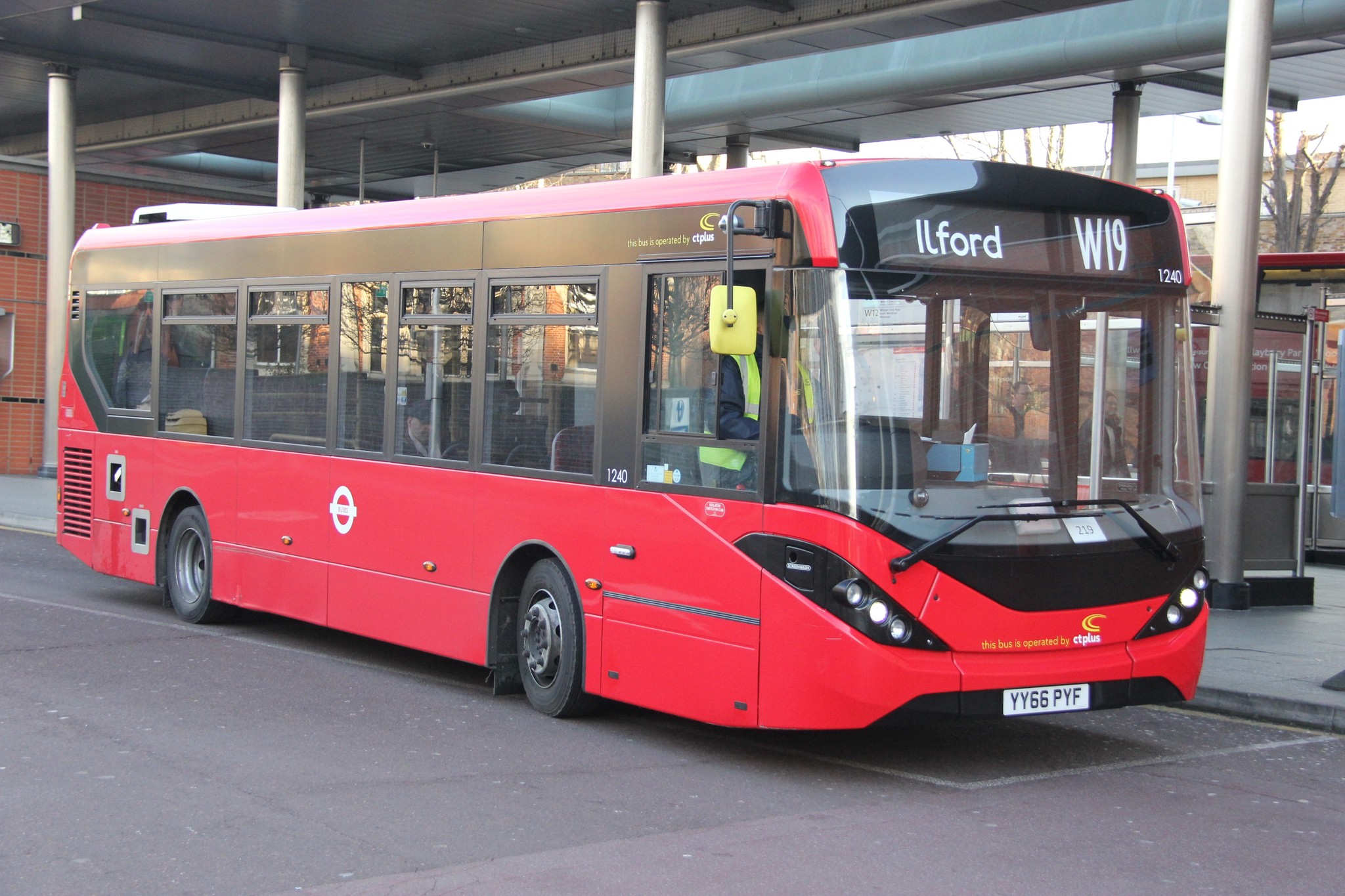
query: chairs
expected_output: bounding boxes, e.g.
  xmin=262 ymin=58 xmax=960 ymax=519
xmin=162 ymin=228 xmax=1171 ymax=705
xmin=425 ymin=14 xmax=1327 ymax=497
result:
xmin=442 ymin=425 xmax=595 ymax=475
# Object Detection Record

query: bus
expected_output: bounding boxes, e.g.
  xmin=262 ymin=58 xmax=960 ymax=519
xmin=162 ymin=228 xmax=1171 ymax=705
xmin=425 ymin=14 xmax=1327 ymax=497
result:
xmin=953 ymin=319 xmax=1336 ymax=489
xmin=52 ymin=157 xmax=1211 ymax=736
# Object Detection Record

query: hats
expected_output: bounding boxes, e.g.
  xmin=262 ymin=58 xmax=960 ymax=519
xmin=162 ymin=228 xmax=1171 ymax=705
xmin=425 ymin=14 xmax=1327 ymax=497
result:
xmin=407 ymin=399 xmax=445 ymax=423
xmin=1104 ymin=390 xmax=1117 ymax=401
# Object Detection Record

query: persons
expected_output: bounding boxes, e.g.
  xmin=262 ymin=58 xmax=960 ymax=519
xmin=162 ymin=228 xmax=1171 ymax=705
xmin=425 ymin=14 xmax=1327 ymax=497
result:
xmin=1003 ymin=381 xmax=1040 ymax=437
xmin=400 ymin=398 xmax=432 ymax=453
xmin=708 ymin=295 xmax=825 ymax=509
xmin=1077 ymin=390 xmax=1132 ymax=480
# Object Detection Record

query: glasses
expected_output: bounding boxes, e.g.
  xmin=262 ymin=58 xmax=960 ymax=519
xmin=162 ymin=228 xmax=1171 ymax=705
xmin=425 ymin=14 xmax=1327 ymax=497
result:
xmin=1015 ymin=393 xmax=1033 ymax=397
